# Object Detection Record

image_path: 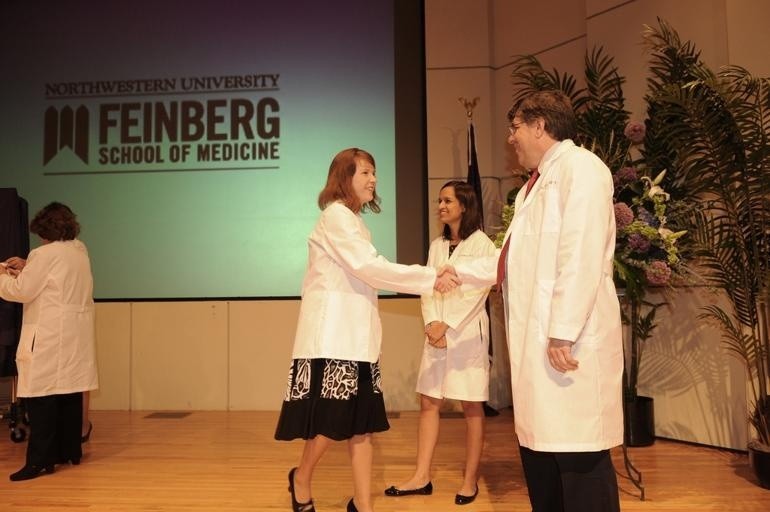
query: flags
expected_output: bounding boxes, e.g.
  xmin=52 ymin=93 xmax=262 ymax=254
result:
xmin=465 ymin=115 xmax=495 ymax=370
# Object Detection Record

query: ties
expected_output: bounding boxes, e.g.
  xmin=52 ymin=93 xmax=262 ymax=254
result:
xmin=497 ymin=170 xmax=540 ymax=293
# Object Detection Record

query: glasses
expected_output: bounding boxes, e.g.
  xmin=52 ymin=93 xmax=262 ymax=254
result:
xmin=508 ymin=117 xmax=537 ymax=136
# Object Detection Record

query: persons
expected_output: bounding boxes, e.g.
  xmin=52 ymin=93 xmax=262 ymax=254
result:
xmin=437 ymin=88 xmax=626 ymax=512
xmin=4 ymin=250 xmax=93 ymax=445
xmin=0 ymin=200 xmax=101 ymax=481
xmin=384 ymin=178 xmax=501 ymax=505
xmin=272 ymin=145 xmax=463 ymax=511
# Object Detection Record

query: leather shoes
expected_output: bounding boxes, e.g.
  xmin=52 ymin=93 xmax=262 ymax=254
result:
xmin=384 ymin=480 xmax=433 ymax=496
xmin=455 ymin=482 xmax=479 ymax=506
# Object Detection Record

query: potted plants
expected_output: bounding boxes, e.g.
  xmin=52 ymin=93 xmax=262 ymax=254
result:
xmin=637 ymin=16 xmax=770 ymax=490
xmin=509 ymin=46 xmax=712 ymax=448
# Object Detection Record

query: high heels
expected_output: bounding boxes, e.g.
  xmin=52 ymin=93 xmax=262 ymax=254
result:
xmin=9 ymin=452 xmax=80 ymax=483
xmin=287 ymin=466 xmax=359 ymax=512
